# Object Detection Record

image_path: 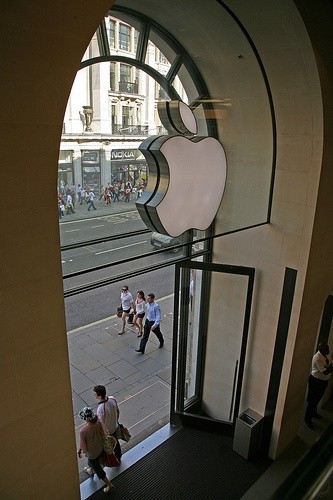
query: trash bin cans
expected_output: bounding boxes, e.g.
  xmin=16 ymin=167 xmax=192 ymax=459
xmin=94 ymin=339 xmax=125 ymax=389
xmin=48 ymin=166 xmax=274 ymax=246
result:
xmin=232 ymin=408 xmax=264 ymax=461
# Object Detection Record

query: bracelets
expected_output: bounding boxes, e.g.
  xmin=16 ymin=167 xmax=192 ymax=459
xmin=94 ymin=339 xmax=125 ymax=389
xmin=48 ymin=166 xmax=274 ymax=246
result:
xmin=78 ymin=452 xmax=81 ymax=454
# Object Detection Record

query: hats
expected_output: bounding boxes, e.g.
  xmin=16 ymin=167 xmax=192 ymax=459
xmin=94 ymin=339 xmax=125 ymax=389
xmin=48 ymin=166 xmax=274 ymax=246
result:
xmin=80 ymin=406 xmax=96 ymax=422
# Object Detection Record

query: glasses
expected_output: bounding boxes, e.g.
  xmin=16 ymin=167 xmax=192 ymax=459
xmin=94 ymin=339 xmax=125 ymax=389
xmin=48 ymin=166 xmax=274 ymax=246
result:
xmin=122 ymin=289 xmax=127 ymax=291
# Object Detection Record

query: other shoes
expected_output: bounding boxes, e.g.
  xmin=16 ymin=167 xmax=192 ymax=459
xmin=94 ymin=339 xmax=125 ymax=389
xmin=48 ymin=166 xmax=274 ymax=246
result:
xmin=118 ymin=331 xmax=125 ymax=336
xmin=313 ymin=412 xmax=322 ymax=420
xmin=83 ymin=466 xmax=94 ymax=477
xmin=131 ymin=324 xmax=135 ymax=327
xmin=305 ymin=419 xmax=316 ymax=429
xmin=94 ymin=208 xmax=98 ymax=210
xmin=88 ymin=209 xmax=91 ymax=211
xmin=159 ymin=340 xmax=164 ymax=348
xmin=137 ymin=331 xmax=143 ymax=338
xmin=136 ymin=348 xmax=145 ymax=355
xmin=67 ymin=213 xmax=70 ymax=215
xmin=103 ymin=484 xmax=115 ymax=493
xmin=73 ymin=212 xmax=76 ymax=213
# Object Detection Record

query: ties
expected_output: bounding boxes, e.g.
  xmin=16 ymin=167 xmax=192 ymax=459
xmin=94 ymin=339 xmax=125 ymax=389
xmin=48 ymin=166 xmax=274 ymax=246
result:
xmin=324 ymin=357 xmax=330 ymax=365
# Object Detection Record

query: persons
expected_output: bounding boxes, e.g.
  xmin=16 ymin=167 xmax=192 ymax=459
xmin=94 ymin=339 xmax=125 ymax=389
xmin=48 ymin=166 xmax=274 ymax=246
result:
xmin=304 ymin=342 xmax=333 ymax=428
xmin=77 ymin=184 xmax=82 ymax=202
xmin=93 ymin=385 xmax=121 ymax=463
xmin=133 ymin=291 xmax=145 ymax=337
xmin=118 ymin=286 xmax=134 ymax=335
xmin=78 ymin=409 xmax=114 ymax=493
xmin=88 ymin=189 xmax=97 ymax=211
xmin=80 ymin=188 xmax=88 ymax=205
xmin=135 ymin=294 xmax=164 ymax=354
xmin=58 ymin=179 xmax=76 ymax=219
xmin=98 ymin=175 xmax=146 ymax=204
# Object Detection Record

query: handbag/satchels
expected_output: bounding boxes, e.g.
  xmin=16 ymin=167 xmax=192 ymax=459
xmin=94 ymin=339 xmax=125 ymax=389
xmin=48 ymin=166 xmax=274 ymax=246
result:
xmin=116 ymin=307 xmax=123 ymax=318
xmin=102 ymin=435 xmax=116 ymax=454
xmin=118 ymin=424 xmax=132 ymax=443
xmin=127 ymin=310 xmax=135 ymax=324
xmin=100 ymin=451 xmax=120 ymax=467
